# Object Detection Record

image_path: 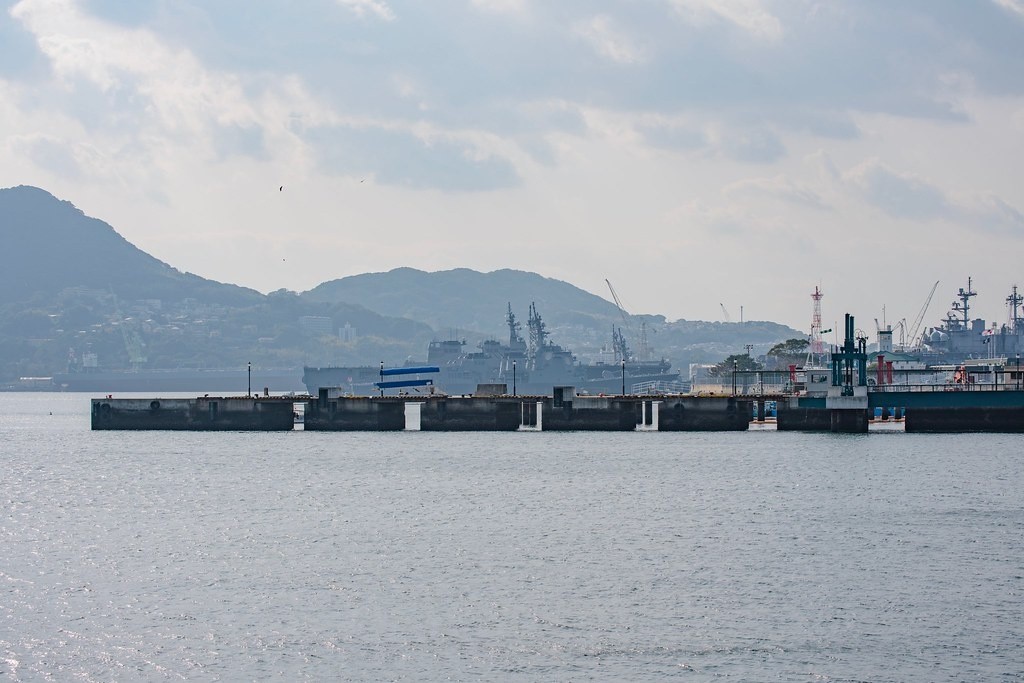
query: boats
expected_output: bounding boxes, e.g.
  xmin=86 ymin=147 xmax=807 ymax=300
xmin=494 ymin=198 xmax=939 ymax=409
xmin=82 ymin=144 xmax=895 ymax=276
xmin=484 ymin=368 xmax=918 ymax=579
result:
xmin=303 ymin=300 xmax=680 ymax=397
xmin=631 ymin=277 xmax=1024 ymax=418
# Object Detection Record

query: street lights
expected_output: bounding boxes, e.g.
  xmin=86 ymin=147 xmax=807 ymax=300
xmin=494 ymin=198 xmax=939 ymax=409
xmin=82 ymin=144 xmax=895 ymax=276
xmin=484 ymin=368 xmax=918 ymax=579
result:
xmin=622 ymin=359 xmax=625 ymax=395
xmin=1016 ymin=354 xmax=1020 ymax=390
xmin=380 ymin=361 xmax=384 ymax=396
xmin=248 ymin=362 xmax=251 ymax=399
xmin=734 ymin=359 xmax=737 ymax=395
xmin=513 ymin=360 xmax=516 ymax=396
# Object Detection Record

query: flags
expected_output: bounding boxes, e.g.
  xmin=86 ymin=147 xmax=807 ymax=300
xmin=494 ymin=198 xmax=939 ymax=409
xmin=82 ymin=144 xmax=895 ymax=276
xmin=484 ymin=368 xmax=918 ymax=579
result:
xmin=983 ymin=338 xmax=991 ymax=344
xmin=979 ymin=329 xmax=994 ymax=336
